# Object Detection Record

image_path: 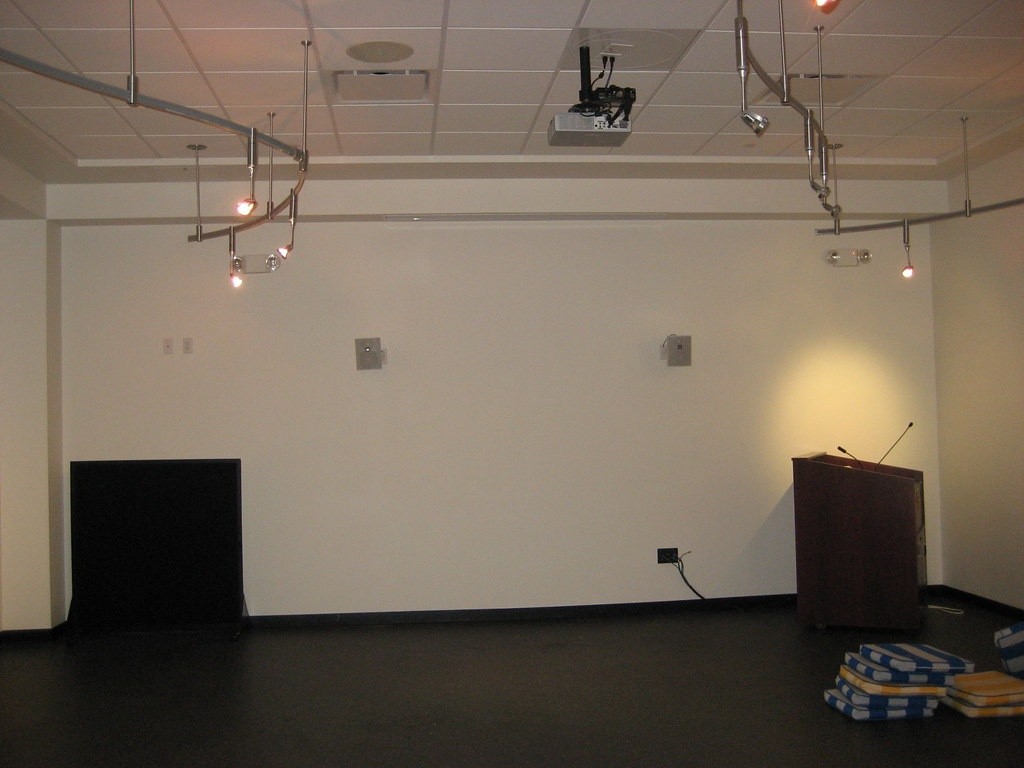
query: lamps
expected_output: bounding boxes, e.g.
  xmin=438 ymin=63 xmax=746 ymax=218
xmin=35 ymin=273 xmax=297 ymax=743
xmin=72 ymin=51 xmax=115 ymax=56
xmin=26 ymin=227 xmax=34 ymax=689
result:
xmin=228 ymin=225 xmax=243 ymax=287
xmin=818 ymin=136 xmax=842 ymax=219
xmin=902 ymin=219 xmax=915 ymax=278
xmin=276 ymin=188 xmax=297 ymax=258
xmin=804 ymin=108 xmax=831 ymax=199
xmin=229 ymin=127 xmax=258 ymax=216
xmin=734 ymin=0 xmax=770 ymax=138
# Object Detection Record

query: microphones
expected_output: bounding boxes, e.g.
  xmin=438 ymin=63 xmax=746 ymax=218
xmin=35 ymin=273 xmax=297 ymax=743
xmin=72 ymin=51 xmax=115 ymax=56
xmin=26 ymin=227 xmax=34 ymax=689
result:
xmin=838 ymin=446 xmax=864 ymax=470
xmin=874 ymin=421 xmax=914 ymax=471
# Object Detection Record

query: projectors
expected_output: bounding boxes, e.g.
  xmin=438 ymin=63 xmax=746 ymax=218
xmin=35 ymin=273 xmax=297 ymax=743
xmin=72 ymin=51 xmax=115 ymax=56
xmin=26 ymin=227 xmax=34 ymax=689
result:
xmin=546 ymin=114 xmax=629 ymax=147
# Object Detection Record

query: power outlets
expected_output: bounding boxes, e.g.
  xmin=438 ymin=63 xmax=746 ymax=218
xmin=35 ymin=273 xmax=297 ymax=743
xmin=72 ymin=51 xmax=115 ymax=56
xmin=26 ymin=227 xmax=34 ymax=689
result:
xmin=164 ymin=337 xmax=173 ymax=354
xmin=183 ymin=337 xmax=194 ymax=354
xmin=657 ymin=548 xmax=679 ymax=564
